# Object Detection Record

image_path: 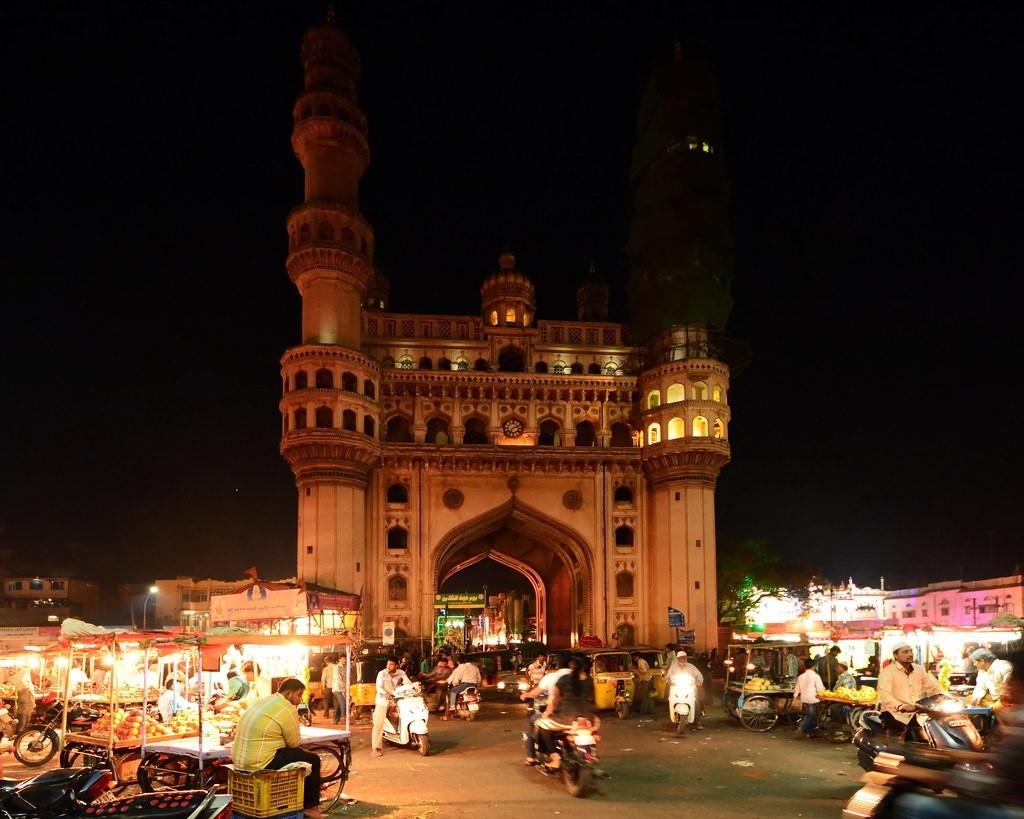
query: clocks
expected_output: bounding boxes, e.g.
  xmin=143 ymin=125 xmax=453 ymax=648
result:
xmin=503 ymin=418 xmax=523 ymax=439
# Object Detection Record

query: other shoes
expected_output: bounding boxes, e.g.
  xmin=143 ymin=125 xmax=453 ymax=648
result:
xmin=697 ymin=722 xmax=703 ymax=730
xmin=325 ymin=716 xmax=330 ymax=718
xmin=523 ymin=758 xmax=538 ymax=766
xmin=439 ymin=716 xmax=448 ymax=721
xmin=304 ymin=808 xmax=328 ymax=819
xmin=341 ymin=721 xmax=345 ymax=724
xmin=449 ymin=705 xmax=456 ymax=710
xmin=794 ymin=730 xmax=803 ymax=740
xmin=545 ymin=757 xmax=562 ymax=769
xmin=371 ymin=751 xmax=382 ymax=757
xmin=333 ymin=722 xmax=338 ymax=725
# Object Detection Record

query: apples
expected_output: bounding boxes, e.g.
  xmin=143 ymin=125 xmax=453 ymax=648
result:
xmin=85 ymin=706 xmax=200 ymax=740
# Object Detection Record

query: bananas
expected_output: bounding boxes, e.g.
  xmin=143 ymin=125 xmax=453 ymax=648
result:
xmin=816 ymin=684 xmax=877 ymax=700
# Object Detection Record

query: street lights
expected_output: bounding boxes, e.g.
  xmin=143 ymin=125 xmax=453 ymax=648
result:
xmin=142 ymin=586 xmax=158 ymax=630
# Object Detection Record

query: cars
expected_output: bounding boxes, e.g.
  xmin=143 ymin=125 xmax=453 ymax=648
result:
xmin=496 ymin=661 xmax=536 ymax=703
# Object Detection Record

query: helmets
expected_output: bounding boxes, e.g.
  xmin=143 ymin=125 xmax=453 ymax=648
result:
xmin=677 ymin=651 xmax=687 ymax=665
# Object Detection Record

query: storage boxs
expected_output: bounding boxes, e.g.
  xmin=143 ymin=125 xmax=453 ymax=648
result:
xmin=228 ymin=769 xmax=305 ymax=819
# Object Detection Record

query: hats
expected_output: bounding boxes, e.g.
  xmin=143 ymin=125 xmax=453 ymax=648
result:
xmin=165 ymin=670 xmax=185 ymax=685
xmin=840 ymin=661 xmax=847 ymax=667
xmin=893 ymin=642 xmax=908 ymax=652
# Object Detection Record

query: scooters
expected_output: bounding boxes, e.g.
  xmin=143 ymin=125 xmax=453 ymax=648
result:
xmin=666 ymin=674 xmax=701 ymax=735
xmin=6 ymin=698 xmax=101 ymax=767
xmin=371 ymin=680 xmax=431 ymax=757
xmin=0 ymin=750 xmax=235 ymax=819
xmin=851 ymin=692 xmax=995 ymax=775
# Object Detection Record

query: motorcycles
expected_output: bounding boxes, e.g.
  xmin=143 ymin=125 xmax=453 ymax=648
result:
xmin=348 ymin=653 xmax=389 ymax=721
xmin=614 ymin=643 xmax=669 ymax=705
xmin=520 ymin=698 xmax=612 ymax=798
xmin=452 ymin=686 xmax=483 ymax=722
xmin=570 ymin=646 xmax=636 ymax=720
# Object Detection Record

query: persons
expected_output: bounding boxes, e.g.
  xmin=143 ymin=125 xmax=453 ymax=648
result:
xmin=927 ymin=642 xmax=1024 ymax=819
xmin=877 ymin=642 xmax=950 ymax=738
xmin=398 ymin=648 xmax=548 ymax=721
xmin=239 ymin=661 xmax=271 ymax=709
xmin=103 ymin=663 xmax=125 ymax=689
xmin=133 ymin=664 xmax=157 ymax=689
xmin=230 ymin=679 xmax=330 ymax=818
xmin=68 ymin=664 xmax=89 ymax=698
xmin=574 ymin=643 xmax=878 ymax=741
xmin=158 ymin=679 xmax=200 ymax=722
xmin=520 ymin=652 xmax=572 ymax=766
xmin=223 ymin=670 xmax=249 ymax=701
xmin=331 ymin=654 xmax=347 ymax=724
xmin=371 ymin=657 xmax=414 ymax=757
xmin=321 ymin=656 xmax=337 ymax=718
xmin=0 ymin=673 xmax=36 ymax=756
xmin=539 ymin=652 xmax=595 ymax=769
